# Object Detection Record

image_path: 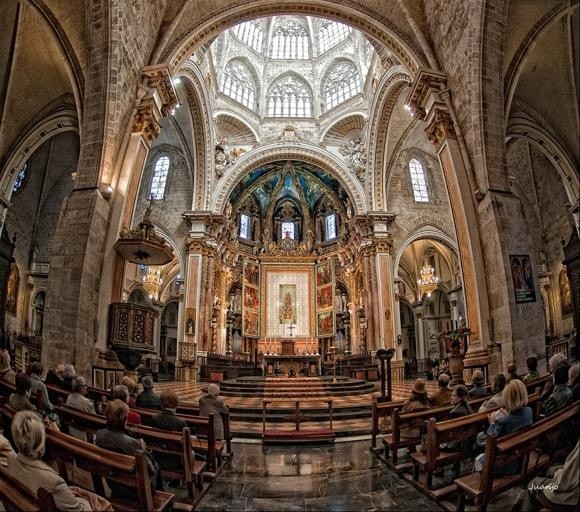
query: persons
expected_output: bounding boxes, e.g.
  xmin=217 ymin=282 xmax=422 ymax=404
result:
xmin=400 ymin=351 xmax=580 ymax=512
xmin=270 ymin=351 xmax=277 ymax=355
xmin=0 ymin=348 xmax=229 ymax=512
xmin=305 ymin=351 xmax=311 ymax=356
xmin=311 ymin=350 xmax=320 ymax=355
xmin=263 ymin=350 xmax=272 ymax=356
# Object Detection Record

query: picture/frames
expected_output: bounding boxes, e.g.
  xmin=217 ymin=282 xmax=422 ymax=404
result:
xmin=313 ymin=256 xmax=338 ymax=339
xmin=507 ymin=252 xmax=537 ymax=305
xmin=241 ymin=258 xmax=262 ymax=339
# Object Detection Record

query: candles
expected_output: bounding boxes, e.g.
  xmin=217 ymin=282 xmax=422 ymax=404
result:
xmin=264 ymin=331 xmax=321 ymax=349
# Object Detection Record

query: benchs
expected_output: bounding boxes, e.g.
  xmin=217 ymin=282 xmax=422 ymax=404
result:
xmin=1 ymin=346 xmax=580 ymax=510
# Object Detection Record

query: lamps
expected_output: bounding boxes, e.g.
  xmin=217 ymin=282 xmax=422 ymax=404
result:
xmin=141 ymin=265 xmax=165 ymax=300
xmin=414 ymin=235 xmax=439 ymax=300
